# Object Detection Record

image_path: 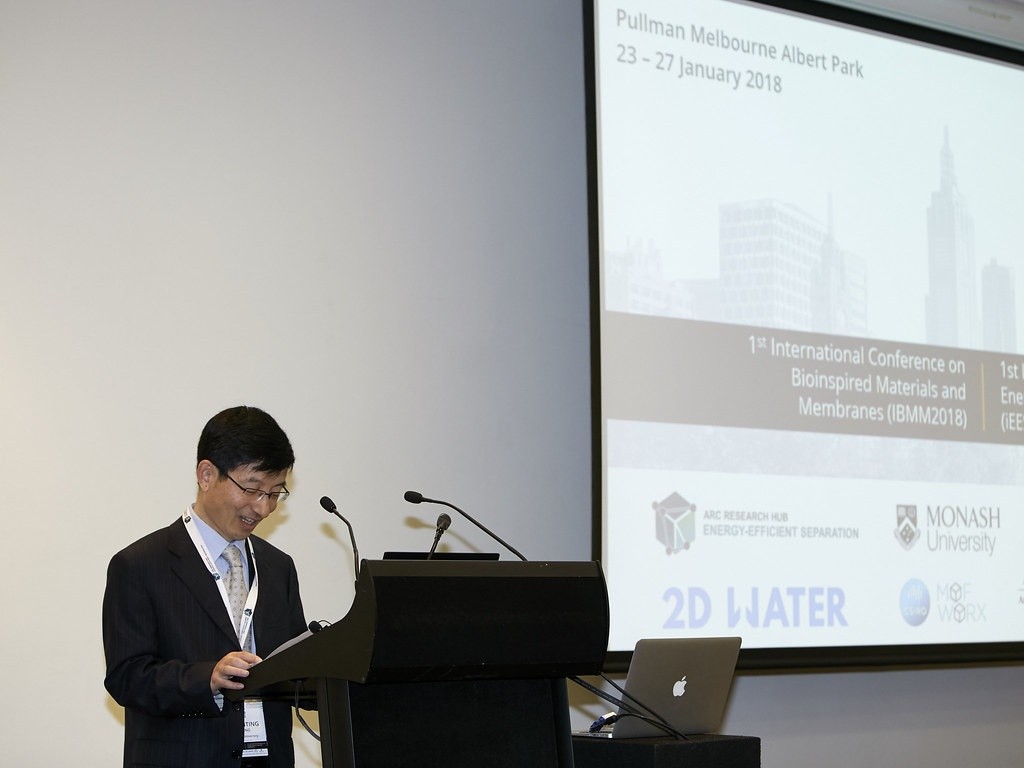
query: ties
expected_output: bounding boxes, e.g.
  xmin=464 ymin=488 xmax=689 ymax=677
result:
xmin=221 ymin=541 xmax=251 ymax=656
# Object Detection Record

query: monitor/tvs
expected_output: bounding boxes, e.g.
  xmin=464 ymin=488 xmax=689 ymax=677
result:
xmin=382 ymin=551 xmax=500 ymax=562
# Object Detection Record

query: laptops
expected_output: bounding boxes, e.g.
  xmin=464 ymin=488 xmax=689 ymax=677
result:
xmin=571 ymin=637 xmax=742 ymax=738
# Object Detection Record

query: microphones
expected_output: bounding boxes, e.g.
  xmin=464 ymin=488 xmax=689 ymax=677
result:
xmin=404 ymin=491 xmax=528 ymax=562
xmin=426 ymin=513 xmax=451 ymax=561
xmin=319 ymin=496 xmax=359 ymax=580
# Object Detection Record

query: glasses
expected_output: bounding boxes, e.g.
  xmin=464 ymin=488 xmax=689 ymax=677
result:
xmin=215 ymin=467 xmax=290 ymax=503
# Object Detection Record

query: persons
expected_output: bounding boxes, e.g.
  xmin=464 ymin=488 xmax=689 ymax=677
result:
xmin=101 ymin=405 xmax=310 ymax=768
